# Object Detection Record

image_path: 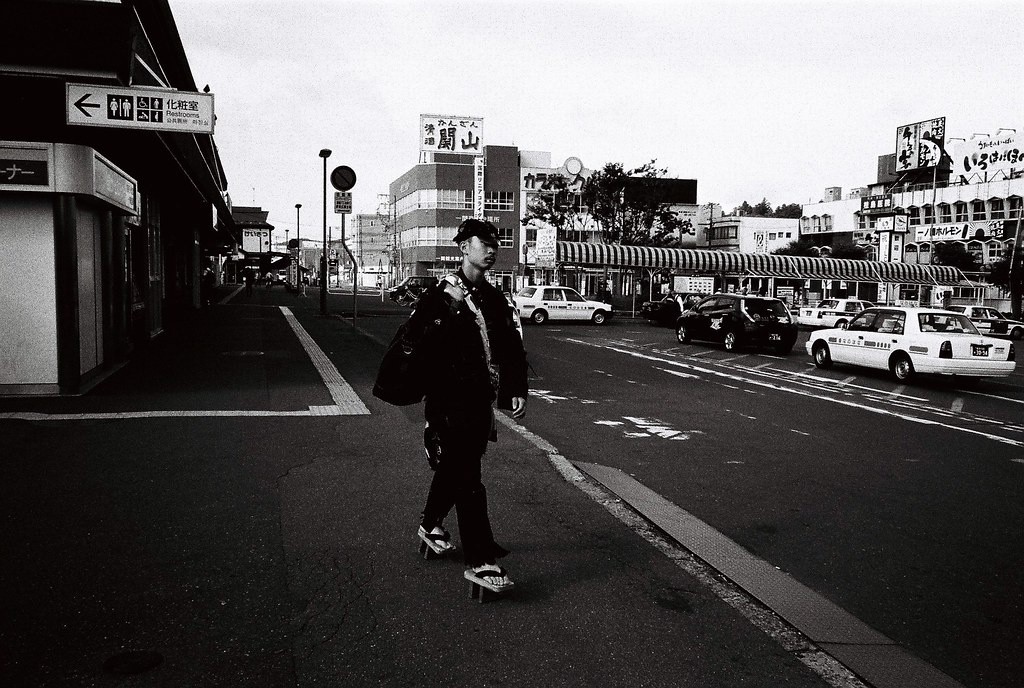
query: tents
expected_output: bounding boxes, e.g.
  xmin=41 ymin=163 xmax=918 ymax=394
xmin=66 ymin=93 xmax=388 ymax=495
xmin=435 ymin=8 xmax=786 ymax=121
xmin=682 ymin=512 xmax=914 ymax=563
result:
xmin=555 ymin=241 xmax=961 ymax=326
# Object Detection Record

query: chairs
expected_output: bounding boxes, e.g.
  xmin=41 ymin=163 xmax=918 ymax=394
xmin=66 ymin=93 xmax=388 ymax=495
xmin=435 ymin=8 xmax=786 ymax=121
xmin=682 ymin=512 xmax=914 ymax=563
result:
xmin=878 ymin=319 xmax=897 ymax=332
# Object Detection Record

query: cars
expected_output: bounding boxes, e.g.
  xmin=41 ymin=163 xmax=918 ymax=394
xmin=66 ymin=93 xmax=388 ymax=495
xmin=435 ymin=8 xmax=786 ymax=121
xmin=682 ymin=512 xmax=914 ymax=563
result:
xmin=674 ymin=293 xmax=798 ymax=355
xmin=641 ymin=292 xmax=715 ymax=330
xmin=505 ymin=284 xmax=614 ymax=326
xmin=923 ymin=304 xmax=1024 ymax=340
xmin=796 ymin=299 xmax=905 ymax=329
xmin=805 ymin=306 xmax=1016 ymax=384
xmin=389 ymin=276 xmax=439 ymax=301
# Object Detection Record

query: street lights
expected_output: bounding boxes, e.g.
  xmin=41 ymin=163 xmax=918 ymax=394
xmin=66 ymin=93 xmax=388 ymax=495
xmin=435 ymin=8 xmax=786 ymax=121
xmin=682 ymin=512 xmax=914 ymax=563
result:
xmin=319 ymin=148 xmax=332 ymax=320
xmin=294 ymin=203 xmax=302 ymax=297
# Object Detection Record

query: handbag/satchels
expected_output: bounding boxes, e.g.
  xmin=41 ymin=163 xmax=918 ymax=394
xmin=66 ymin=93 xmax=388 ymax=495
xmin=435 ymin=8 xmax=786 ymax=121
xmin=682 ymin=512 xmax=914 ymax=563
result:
xmin=372 ymin=279 xmax=448 ymax=406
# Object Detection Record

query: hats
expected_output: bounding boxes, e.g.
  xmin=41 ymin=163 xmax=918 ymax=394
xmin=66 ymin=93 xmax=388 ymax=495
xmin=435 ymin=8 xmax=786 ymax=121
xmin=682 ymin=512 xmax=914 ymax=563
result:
xmin=453 ymin=219 xmax=501 ymax=246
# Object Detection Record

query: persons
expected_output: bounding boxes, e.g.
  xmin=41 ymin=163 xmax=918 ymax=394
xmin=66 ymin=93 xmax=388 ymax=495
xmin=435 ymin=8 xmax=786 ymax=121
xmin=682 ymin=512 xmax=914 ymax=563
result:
xmin=416 ymin=218 xmax=529 ymax=594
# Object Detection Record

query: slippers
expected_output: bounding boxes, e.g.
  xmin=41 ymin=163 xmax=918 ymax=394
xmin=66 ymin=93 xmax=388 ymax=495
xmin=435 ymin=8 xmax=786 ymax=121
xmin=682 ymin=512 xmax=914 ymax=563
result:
xmin=464 ymin=567 xmax=515 ymax=604
xmin=417 ymin=528 xmax=456 ymax=559
